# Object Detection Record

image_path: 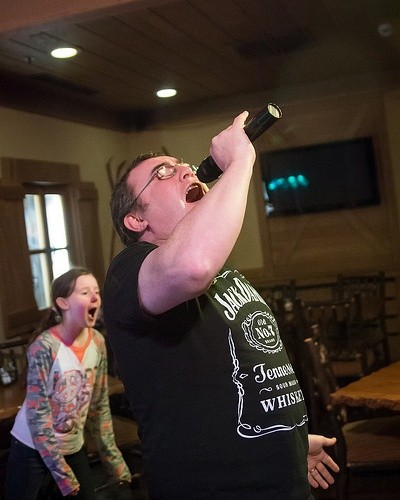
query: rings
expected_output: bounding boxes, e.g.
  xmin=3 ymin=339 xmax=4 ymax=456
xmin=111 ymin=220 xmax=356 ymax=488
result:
xmin=309 ymin=468 xmax=315 ymax=473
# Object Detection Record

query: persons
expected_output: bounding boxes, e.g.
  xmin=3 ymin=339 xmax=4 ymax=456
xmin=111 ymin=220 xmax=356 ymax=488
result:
xmin=6 ymin=268 xmax=132 ymax=500
xmin=101 ymin=110 xmax=341 ymax=500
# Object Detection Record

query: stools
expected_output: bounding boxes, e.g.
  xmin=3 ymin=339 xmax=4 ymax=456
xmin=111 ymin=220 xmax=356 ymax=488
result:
xmin=325 ymin=417 xmax=400 ymax=500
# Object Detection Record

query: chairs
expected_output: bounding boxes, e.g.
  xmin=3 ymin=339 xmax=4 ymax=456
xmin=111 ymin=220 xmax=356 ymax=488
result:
xmin=252 ymin=269 xmax=390 ymax=434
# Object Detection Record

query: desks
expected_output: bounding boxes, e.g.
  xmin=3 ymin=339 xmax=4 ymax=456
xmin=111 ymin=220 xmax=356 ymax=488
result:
xmin=0 ymin=375 xmax=125 ymax=420
xmin=329 ymin=360 xmax=400 ymax=421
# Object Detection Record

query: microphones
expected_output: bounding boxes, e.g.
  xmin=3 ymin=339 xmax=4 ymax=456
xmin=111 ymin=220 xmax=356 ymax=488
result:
xmin=196 ymin=103 xmax=282 ymax=183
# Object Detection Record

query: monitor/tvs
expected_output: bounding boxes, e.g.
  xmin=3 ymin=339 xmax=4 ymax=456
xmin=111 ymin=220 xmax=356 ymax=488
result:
xmin=259 ymin=136 xmax=381 ymax=218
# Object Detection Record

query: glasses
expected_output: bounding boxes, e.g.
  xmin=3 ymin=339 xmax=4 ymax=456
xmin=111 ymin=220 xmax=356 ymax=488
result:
xmin=125 ymin=163 xmax=198 ymax=217
